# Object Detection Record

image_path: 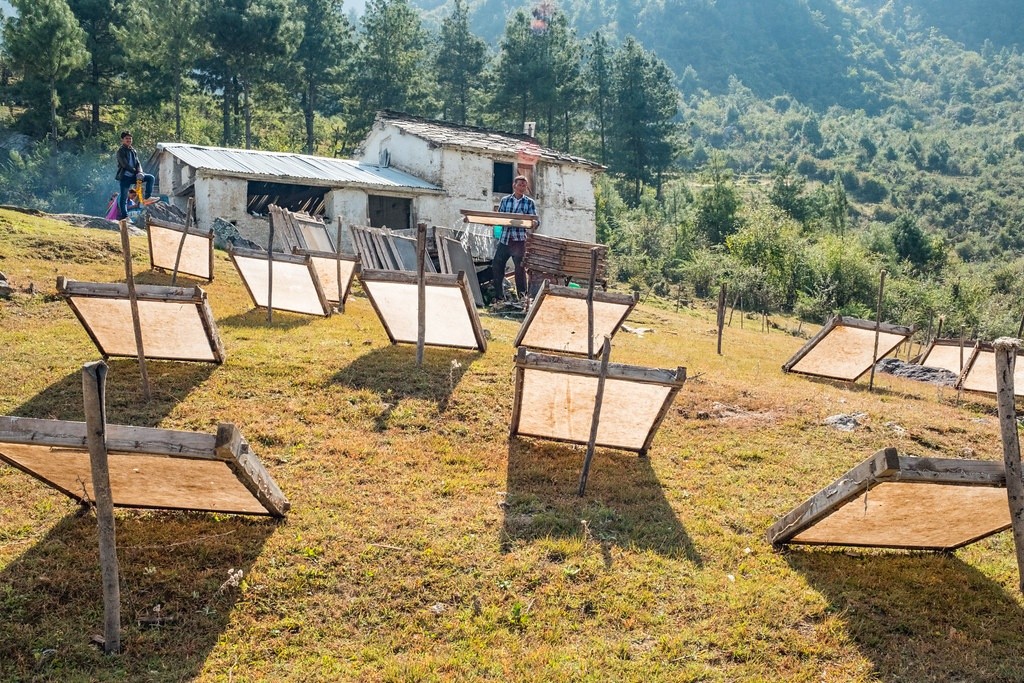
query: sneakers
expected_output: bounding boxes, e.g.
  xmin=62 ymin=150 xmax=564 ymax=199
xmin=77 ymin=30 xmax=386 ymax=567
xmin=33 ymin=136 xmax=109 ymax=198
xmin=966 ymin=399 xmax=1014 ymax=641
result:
xmin=144 ymin=196 xmax=160 ymax=205
xmin=116 ymin=215 xmax=133 ymax=223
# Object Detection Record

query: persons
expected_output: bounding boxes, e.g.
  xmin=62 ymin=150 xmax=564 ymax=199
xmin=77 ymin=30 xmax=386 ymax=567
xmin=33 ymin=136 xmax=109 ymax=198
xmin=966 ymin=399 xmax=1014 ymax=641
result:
xmin=114 ymin=131 xmax=160 ymax=224
xmin=108 ymin=192 xmax=118 ymax=206
xmin=115 ymin=189 xmax=139 ymax=221
xmin=484 ymin=175 xmax=540 ymax=302
